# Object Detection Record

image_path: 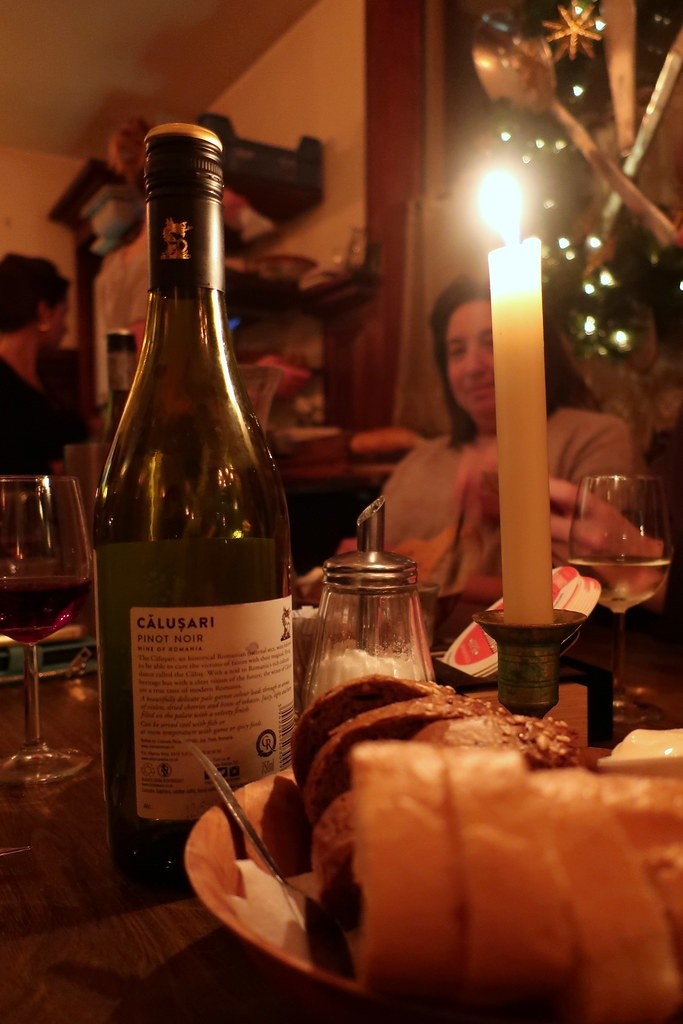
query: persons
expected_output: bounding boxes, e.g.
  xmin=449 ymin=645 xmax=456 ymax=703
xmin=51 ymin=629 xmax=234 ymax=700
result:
xmin=0 ymin=253 xmax=93 ymax=474
xmin=480 ymin=460 xmax=683 ymax=619
xmin=296 ymin=285 xmax=635 ymax=641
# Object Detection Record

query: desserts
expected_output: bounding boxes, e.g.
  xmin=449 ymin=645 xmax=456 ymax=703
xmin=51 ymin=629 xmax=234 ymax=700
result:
xmin=261 ymin=675 xmax=682 ymax=1024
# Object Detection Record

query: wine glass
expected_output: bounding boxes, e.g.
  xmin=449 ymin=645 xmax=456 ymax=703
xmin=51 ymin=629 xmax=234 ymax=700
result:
xmin=566 ymin=477 xmax=672 ymax=724
xmin=0 ymin=475 xmax=100 ymax=787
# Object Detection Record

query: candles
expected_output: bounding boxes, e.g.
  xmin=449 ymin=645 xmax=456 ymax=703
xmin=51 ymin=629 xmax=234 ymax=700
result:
xmin=474 ymin=159 xmax=560 ymax=628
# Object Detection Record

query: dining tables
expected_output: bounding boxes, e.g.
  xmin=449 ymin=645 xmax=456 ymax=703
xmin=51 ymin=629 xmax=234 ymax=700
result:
xmin=0 ymin=652 xmax=683 ymax=1024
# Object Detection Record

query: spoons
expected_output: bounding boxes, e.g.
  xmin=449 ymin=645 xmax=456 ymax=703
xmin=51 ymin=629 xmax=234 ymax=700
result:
xmin=188 ymin=745 xmax=358 ymax=985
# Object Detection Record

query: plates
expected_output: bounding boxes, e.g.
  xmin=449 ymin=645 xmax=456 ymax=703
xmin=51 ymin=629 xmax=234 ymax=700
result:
xmin=185 ymin=771 xmax=361 ymax=995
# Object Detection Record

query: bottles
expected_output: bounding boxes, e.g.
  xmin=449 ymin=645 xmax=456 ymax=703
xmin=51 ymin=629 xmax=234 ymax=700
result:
xmin=102 ymin=331 xmax=137 ymax=471
xmin=301 ymin=497 xmax=435 ymax=711
xmin=90 ymin=124 xmax=289 ymax=904
